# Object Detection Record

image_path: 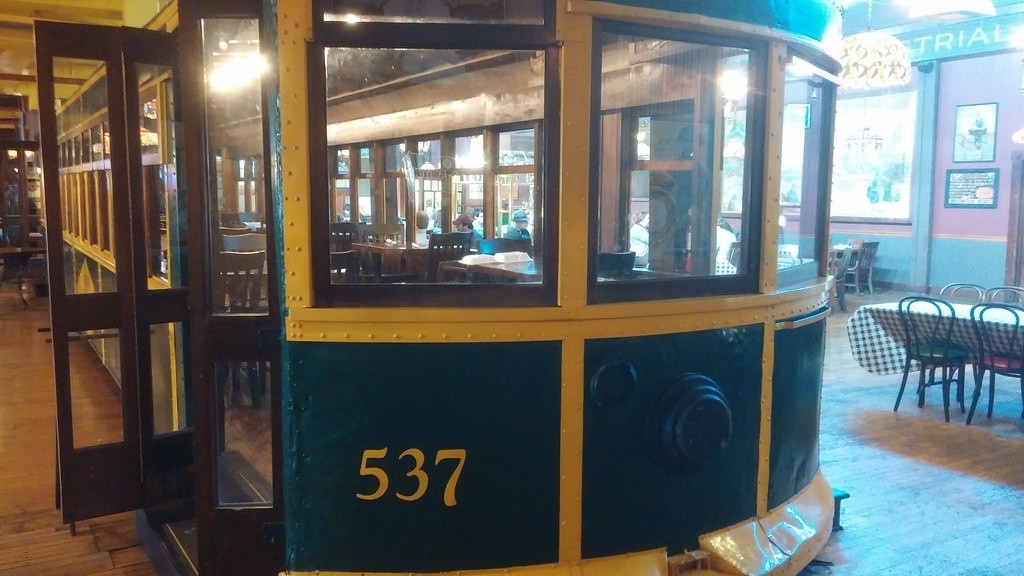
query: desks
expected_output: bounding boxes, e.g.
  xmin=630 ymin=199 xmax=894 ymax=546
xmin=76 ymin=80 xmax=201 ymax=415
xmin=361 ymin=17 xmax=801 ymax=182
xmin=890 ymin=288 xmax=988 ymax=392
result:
xmin=218 ymin=260 xmax=346 ymax=313
xmin=352 ymin=240 xmax=463 ymax=274
xmin=438 ymin=260 xmax=543 ymax=283
xmin=0 ymin=247 xmax=47 ymax=309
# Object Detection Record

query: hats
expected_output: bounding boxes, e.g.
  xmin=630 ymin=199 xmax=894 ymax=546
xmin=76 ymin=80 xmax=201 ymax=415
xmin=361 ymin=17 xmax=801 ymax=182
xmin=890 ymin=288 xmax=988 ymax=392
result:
xmin=453 ymin=214 xmax=474 ymax=225
xmin=511 ymin=210 xmax=529 ymax=221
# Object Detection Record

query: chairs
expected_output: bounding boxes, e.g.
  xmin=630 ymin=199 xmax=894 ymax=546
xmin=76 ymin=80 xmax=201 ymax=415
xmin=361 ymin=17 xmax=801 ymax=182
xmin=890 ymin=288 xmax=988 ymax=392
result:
xmin=828 ymin=239 xmax=1023 ymax=424
xmin=716 ymin=242 xmax=799 ymax=275
xmin=218 ymin=217 xmax=636 ymax=408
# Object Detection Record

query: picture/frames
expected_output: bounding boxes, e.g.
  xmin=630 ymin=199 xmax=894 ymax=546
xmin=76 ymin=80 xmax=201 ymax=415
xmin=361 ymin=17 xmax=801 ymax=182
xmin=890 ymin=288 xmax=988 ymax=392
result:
xmin=953 ymin=102 xmax=998 ymax=163
xmin=944 ymin=168 xmax=999 ymax=208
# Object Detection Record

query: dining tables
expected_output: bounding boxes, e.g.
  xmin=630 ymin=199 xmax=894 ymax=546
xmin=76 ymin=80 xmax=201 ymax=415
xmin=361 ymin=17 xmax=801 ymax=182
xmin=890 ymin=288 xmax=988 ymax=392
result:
xmin=846 ymin=300 xmax=1024 ymax=407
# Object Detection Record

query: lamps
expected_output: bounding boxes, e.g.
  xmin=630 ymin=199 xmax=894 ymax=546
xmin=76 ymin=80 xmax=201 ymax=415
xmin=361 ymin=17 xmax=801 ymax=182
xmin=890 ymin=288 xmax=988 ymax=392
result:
xmin=840 ymin=0 xmax=910 ymax=89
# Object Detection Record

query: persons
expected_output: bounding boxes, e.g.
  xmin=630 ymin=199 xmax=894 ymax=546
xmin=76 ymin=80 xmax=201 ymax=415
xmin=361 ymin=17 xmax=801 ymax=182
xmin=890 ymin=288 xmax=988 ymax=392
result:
xmin=414 ymin=200 xmax=484 ymax=249
xmin=506 ymin=211 xmax=529 ymax=238
xmin=628 ymin=211 xmax=649 ymax=264
xmin=338 ymin=204 xmax=365 ymax=223
